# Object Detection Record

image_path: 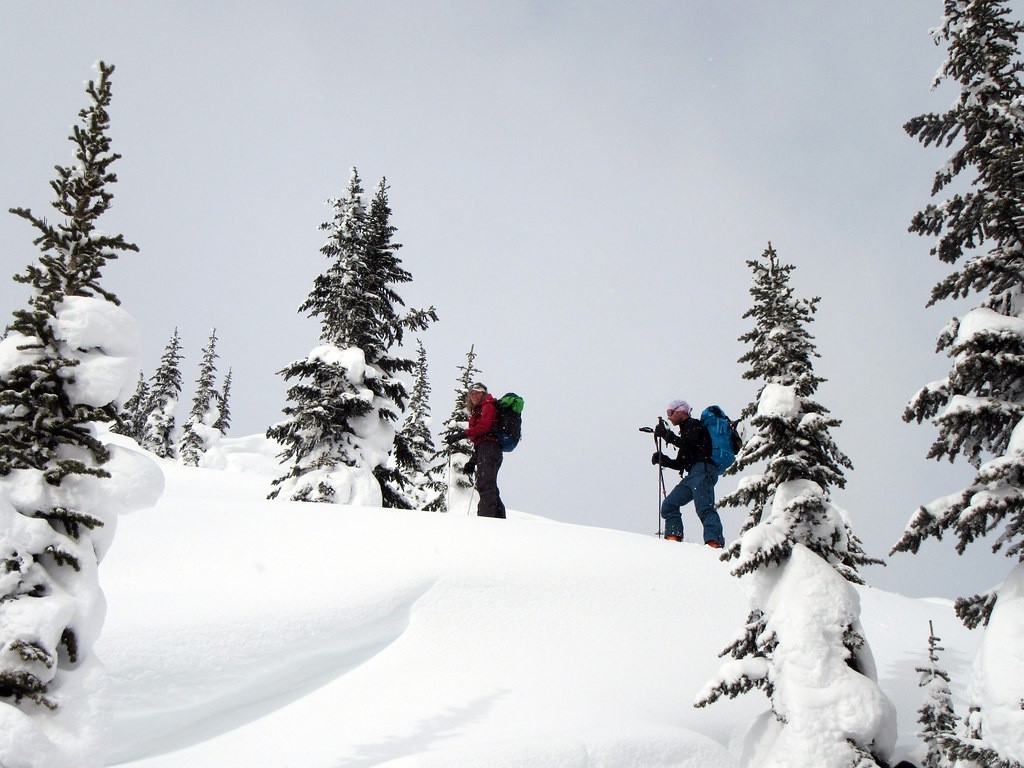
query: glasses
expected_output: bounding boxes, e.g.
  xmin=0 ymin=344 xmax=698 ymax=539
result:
xmin=668 ymin=404 xmax=682 ymax=416
xmin=468 ymin=384 xmax=486 ymax=393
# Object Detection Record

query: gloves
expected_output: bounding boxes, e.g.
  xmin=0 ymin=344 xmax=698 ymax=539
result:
xmin=463 ymin=461 xmax=475 ymax=475
xmin=655 ymin=423 xmax=668 ymax=438
xmin=444 ymin=433 xmax=466 ymax=445
xmin=652 ymin=451 xmax=670 ymax=468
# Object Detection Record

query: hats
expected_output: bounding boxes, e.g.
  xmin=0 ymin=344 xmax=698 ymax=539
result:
xmin=667 ymin=400 xmax=692 ymax=415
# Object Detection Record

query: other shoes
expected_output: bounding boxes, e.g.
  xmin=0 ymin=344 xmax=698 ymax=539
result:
xmin=665 ymin=535 xmax=682 ymax=542
xmin=706 ymin=540 xmax=724 ymax=548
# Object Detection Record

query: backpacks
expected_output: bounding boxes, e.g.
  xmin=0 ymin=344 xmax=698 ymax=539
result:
xmin=686 ymin=405 xmax=742 ymax=475
xmin=475 ymin=392 xmax=524 ymax=452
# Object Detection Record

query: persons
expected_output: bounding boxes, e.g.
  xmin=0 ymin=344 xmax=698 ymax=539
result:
xmin=445 ymin=382 xmax=507 ymax=519
xmin=651 ymin=399 xmax=725 ymax=549
